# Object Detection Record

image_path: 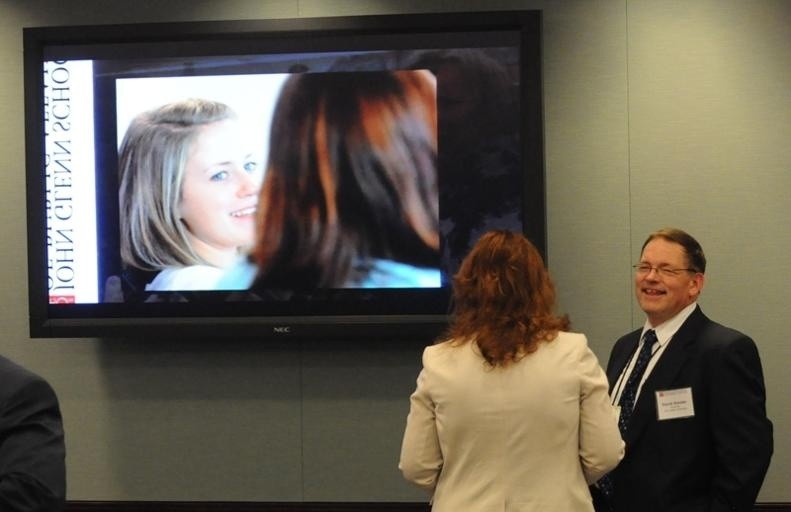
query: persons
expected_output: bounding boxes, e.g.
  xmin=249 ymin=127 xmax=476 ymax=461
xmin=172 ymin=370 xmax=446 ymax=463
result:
xmin=394 ymin=223 xmax=627 ymax=511
xmin=225 ymin=72 xmax=443 ymax=289
xmin=2 ymin=353 xmax=68 ymax=512
xmin=115 ymin=93 xmax=251 ymax=290
xmin=591 ymin=227 xmax=776 ymax=510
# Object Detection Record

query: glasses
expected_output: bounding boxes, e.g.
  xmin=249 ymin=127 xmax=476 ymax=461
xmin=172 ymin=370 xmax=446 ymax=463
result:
xmin=631 ymin=263 xmax=701 ymax=277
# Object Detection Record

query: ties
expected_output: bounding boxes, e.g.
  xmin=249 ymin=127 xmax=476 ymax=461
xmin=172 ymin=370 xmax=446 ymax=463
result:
xmin=594 ymin=328 xmax=660 ymax=497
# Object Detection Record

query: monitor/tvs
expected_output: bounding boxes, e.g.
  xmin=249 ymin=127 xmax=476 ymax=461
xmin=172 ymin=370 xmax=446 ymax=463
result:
xmin=21 ymin=8 xmax=547 ymax=340
